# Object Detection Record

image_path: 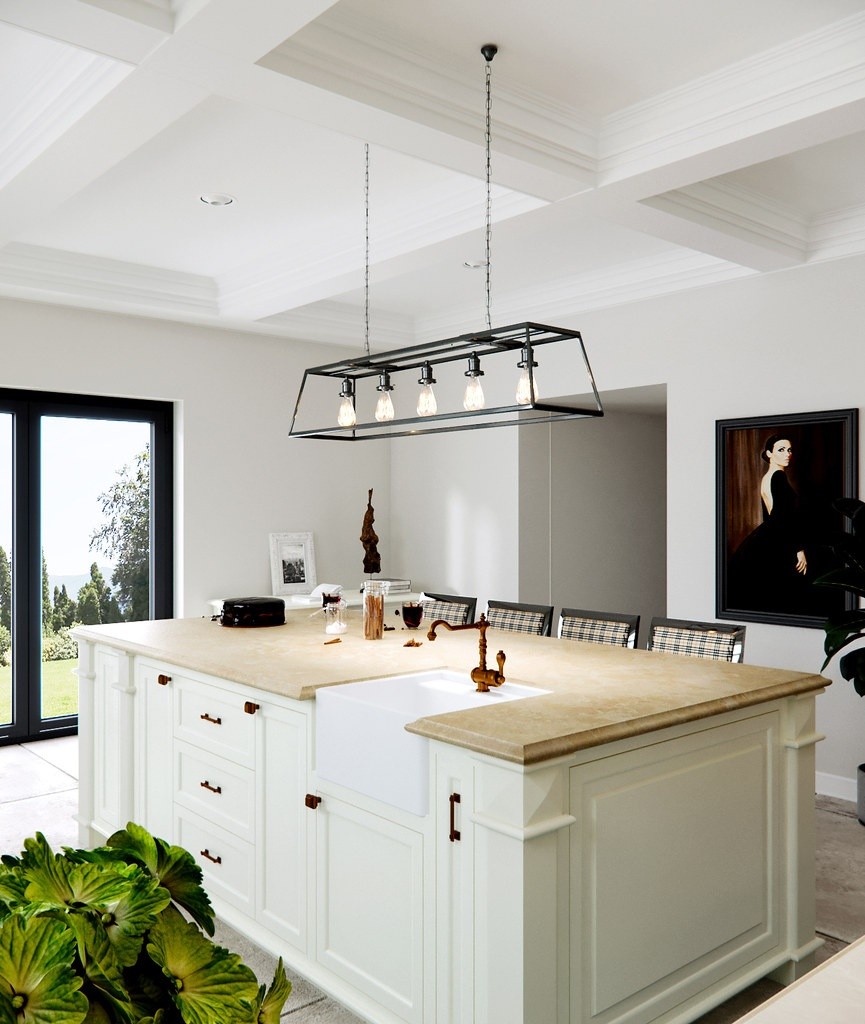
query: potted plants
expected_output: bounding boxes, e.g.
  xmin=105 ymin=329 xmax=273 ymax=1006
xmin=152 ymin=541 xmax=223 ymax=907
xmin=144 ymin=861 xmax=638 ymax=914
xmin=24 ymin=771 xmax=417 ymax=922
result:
xmin=808 ymin=496 xmax=865 ymax=827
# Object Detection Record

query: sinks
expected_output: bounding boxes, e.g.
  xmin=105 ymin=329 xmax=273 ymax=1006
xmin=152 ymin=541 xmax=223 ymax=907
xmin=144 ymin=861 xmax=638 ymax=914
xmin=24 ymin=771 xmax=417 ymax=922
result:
xmin=314 ymin=676 xmax=493 ymax=817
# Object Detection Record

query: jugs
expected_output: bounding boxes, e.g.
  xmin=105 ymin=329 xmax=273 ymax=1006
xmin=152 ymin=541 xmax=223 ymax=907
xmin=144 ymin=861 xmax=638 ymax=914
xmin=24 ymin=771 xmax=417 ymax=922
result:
xmin=363 ymin=580 xmax=390 ymax=640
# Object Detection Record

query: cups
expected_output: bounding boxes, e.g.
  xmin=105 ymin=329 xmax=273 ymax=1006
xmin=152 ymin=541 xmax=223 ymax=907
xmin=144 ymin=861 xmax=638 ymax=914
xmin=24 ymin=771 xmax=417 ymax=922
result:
xmin=401 ymin=601 xmax=424 ymax=629
xmin=321 ymin=591 xmax=341 ymax=616
xmin=325 ymin=603 xmax=347 ymax=635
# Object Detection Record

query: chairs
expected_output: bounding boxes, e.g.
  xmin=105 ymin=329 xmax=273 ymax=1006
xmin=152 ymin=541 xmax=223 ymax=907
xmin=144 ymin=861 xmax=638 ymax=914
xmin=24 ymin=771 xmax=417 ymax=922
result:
xmin=420 ymin=593 xmax=746 ymax=663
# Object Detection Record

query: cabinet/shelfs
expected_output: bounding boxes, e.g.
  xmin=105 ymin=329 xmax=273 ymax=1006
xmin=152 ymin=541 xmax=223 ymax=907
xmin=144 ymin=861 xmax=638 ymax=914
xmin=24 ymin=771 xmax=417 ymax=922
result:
xmin=88 ymin=645 xmax=524 ymax=1024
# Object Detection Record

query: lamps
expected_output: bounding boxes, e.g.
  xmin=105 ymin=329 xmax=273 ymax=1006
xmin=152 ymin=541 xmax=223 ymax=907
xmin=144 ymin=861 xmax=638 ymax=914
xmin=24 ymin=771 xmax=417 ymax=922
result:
xmin=289 ymin=43 xmax=607 ymax=442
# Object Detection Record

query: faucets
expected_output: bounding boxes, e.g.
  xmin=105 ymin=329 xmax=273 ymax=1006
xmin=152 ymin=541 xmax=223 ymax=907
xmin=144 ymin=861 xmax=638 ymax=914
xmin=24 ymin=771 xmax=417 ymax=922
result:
xmin=426 ymin=612 xmax=507 ymax=693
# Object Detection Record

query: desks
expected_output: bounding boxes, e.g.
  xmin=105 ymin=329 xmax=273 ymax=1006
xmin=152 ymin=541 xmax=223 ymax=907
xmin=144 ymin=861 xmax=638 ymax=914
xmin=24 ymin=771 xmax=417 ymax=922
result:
xmin=207 ymin=588 xmax=421 ymax=618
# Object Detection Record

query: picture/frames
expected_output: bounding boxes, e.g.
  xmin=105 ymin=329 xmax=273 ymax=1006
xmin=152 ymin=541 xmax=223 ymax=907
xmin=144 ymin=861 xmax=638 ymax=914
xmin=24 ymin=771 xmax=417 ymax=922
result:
xmin=270 ymin=531 xmax=317 ymax=596
xmin=715 ymin=408 xmax=860 ymax=633
